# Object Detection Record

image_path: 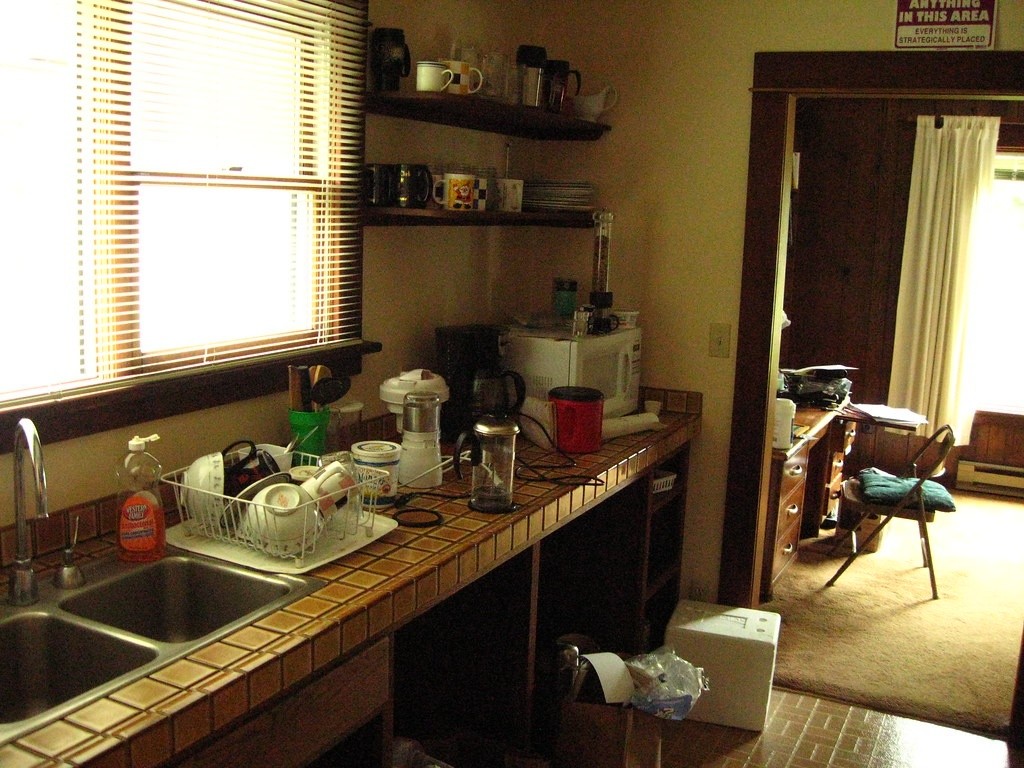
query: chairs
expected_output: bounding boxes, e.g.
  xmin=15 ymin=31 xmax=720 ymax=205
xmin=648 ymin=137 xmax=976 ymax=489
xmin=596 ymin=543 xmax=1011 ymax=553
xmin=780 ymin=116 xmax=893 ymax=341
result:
xmin=825 ymin=426 xmax=957 ymax=599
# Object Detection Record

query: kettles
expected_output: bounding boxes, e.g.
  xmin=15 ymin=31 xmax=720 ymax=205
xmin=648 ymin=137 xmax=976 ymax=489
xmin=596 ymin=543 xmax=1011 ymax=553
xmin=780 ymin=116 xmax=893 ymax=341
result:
xmin=460 ymin=362 xmax=525 ymax=423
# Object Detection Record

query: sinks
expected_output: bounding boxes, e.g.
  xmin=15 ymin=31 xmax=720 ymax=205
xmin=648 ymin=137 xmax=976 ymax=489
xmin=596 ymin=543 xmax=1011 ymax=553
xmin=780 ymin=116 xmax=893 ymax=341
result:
xmin=0 ymin=614 xmax=159 ymax=725
xmin=61 ymin=559 xmax=290 ymax=642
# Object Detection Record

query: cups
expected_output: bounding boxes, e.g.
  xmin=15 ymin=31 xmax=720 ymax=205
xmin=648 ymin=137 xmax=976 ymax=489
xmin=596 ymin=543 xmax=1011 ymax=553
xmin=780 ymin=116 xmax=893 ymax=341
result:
xmin=321 ymin=450 xmax=362 ymax=517
xmin=594 ymin=313 xmax=619 ymax=333
xmin=366 ymin=163 xmax=523 ymax=211
xmin=289 ymin=405 xmax=330 ymax=465
xmin=372 ymin=26 xmax=618 ymax=123
xmin=454 ymin=413 xmax=519 ymax=512
xmin=301 ymin=464 xmax=358 ymax=518
xmin=221 ymin=441 xmax=279 ymax=491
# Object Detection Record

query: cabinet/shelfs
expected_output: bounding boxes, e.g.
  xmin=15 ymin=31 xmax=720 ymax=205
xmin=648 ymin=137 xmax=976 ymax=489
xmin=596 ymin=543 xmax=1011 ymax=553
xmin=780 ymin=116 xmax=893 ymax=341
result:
xmin=152 ymin=441 xmax=692 ymax=768
xmin=758 ymin=396 xmax=856 ymax=604
xmin=362 ymin=89 xmax=612 ymax=229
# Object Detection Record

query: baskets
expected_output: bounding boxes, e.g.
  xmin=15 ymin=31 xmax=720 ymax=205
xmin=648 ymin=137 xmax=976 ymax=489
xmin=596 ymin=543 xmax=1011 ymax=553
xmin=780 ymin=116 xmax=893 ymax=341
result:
xmin=160 ymin=445 xmax=389 ymax=568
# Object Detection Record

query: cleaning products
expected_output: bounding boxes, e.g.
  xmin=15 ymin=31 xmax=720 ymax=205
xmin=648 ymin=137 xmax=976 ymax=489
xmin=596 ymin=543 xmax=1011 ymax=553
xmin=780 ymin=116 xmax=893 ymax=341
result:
xmin=115 ymin=434 xmax=166 ymax=562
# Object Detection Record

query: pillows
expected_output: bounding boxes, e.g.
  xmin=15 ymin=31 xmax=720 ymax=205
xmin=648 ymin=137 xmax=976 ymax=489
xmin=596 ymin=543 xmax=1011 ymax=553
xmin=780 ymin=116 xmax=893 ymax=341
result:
xmin=858 ymin=468 xmax=955 ymax=513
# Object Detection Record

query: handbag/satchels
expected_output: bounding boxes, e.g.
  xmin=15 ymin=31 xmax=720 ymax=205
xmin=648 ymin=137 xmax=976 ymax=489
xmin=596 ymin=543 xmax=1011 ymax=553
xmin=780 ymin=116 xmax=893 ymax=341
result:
xmin=554 ymin=694 xmax=663 ymax=768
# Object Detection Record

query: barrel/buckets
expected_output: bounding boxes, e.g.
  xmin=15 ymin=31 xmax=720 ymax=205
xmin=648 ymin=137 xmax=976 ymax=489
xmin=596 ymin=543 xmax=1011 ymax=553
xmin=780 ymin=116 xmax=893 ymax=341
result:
xmin=549 ymin=388 xmax=605 ymax=452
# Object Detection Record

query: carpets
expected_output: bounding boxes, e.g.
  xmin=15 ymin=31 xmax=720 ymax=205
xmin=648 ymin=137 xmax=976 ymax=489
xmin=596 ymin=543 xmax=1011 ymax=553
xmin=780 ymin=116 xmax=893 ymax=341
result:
xmin=753 ymin=486 xmax=1024 ymax=740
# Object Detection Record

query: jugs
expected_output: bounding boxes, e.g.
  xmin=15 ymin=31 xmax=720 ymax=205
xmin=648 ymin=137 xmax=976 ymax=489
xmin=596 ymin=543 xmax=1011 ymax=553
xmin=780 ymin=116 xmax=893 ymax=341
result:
xmin=451 ymin=366 xmax=525 ymax=415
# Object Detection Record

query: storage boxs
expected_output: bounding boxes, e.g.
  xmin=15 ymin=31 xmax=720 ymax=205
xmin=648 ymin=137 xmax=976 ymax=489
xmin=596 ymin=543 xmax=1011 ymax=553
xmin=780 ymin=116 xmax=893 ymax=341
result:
xmin=664 ymin=598 xmax=781 ymax=731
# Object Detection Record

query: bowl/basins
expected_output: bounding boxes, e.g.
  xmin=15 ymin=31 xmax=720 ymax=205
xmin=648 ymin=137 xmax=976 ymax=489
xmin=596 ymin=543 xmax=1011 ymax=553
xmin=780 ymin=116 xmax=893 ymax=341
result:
xmin=240 ymin=482 xmax=324 ymax=555
xmin=237 ymin=445 xmax=293 ymax=474
xmin=180 ymin=453 xmax=223 ymax=522
xmin=613 ymin=311 xmax=638 ymax=328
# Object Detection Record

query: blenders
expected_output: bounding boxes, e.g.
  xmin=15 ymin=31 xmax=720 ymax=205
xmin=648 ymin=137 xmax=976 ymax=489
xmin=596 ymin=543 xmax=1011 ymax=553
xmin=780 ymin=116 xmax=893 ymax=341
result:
xmin=381 ymin=367 xmax=449 ymax=444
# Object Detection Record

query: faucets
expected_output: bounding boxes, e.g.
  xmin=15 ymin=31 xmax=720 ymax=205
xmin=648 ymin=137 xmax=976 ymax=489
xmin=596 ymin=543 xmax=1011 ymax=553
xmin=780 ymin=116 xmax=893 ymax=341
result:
xmin=10 ymin=418 xmax=50 ymax=606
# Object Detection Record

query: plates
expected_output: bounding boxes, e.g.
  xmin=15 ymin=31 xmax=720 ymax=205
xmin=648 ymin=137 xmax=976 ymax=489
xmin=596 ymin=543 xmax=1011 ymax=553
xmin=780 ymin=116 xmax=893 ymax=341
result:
xmin=220 ymin=474 xmax=291 ymax=527
xmin=523 ymin=178 xmax=595 ymax=211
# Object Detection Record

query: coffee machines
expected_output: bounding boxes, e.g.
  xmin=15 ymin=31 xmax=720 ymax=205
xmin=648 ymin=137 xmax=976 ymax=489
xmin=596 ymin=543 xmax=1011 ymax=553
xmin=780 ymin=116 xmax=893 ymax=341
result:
xmin=436 ymin=324 xmax=506 ymax=420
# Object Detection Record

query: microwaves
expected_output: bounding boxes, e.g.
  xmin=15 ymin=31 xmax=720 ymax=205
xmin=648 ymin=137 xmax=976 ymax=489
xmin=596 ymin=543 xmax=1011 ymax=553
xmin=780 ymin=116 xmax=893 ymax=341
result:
xmin=502 ymin=322 xmax=640 ymax=423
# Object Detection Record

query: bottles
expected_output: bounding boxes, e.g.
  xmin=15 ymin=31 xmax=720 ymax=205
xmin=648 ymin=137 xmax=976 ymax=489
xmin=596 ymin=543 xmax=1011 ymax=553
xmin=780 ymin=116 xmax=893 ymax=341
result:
xmin=591 ymin=291 xmax=612 ymax=323
xmin=773 ymin=397 xmax=796 ymax=450
